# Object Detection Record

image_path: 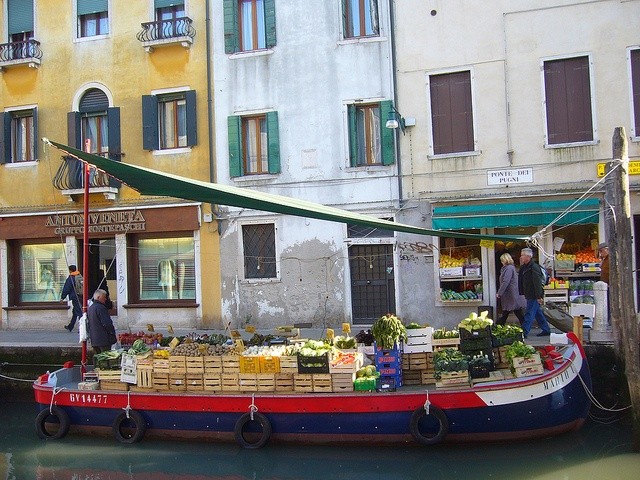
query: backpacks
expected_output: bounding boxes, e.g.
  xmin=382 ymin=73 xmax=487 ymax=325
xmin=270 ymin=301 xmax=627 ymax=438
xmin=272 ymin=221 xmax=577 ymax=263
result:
xmin=75 ymin=274 xmax=83 ymax=295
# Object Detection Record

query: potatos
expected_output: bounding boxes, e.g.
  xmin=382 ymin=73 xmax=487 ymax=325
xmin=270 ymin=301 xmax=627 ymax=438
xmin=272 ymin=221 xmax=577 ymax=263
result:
xmin=170 ymin=342 xmax=231 ymax=357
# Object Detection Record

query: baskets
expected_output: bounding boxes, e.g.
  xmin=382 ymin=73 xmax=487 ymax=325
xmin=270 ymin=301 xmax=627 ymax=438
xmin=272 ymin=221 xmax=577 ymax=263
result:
xmin=492 ymin=333 xmax=523 ymax=347
xmin=469 ymin=363 xmax=489 ymax=377
xmin=460 ymin=325 xmax=490 ymax=339
xmin=93 ymin=357 xmax=121 ymax=369
xmin=297 ymin=352 xmax=328 ymax=373
xmin=467 ymin=350 xmax=494 ymax=371
xmin=376 ymin=379 xmax=395 ymax=392
xmin=355 ymin=380 xmax=374 ymax=390
xmin=460 ymin=339 xmax=492 ymax=350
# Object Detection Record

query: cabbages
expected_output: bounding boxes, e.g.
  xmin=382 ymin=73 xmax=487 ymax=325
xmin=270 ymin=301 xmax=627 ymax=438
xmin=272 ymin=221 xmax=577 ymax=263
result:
xmin=458 ymin=310 xmax=493 ymax=330
xmin=298 ymin=337 xmax=331 ymax=367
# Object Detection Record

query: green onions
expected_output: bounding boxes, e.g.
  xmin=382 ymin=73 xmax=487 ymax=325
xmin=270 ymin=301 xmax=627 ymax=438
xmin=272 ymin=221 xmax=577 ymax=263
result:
xmin=96 ymin=349 xmax=119 ymax=361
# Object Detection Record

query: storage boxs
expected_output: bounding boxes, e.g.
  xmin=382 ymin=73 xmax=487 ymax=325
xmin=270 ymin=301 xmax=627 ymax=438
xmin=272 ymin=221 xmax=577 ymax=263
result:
xmin=99 ymin=359 xmax=121 ymax=370
xmin=374 ymin=341 xmax=402 ymax=353
xmin=516 ymin=364 xmax=543 ymax=377
xmin=204 ymin=356 xmax=222 ymax=373
xmin=330 ymin=354 xmax=363 ymax=373
xmin=257 ymin=374 xmax=275 ymax=391
xmin=465 ymin=268 xmax=480 ymax=277
xmin=186 ymin=375 xmax=203 ymax=391
xmin=402 ymin=353 xmax=434 ymax=370
xmin=137 ymin=366 xmax=152 ymax=387
xmin=280 ymin=356 xmax=298 ymax=373
xmin=239 ymin=374 xmax=257 ymax=392
xmin=582 ymin=262 xmax=602 ymax=271
xmin=433 ymin=335 xmax=460 ymax=353
xmin=401 ymin=370 xmax=435 ymax=385
xmin=313 ymin=373 xmax=332 ymax=392
xmin=375 ymin=366 xmax=401 ymax=378
xmin=100 ymin=380 xmax=128 ymax=391
xmin=355 ymin=377 xmax=376 ymax=391
xmin=130 ymin=386 xmax=156 ymax=393
xmin=169 ymin=356 xmax=186 ymax=373
xmin=333 ymin=374 xmax=356 ymax=393
xmin=513 ymin=351 xmax=540 ymax=368
xmin=402 ymin=328 xmax=432 ymax=354
xmin=543 ymin=289 xmax=568 ymax=307
xmin=169 ymin=375 xmax=186 ymax=392
xmin=376 ymin=378 xmax=401 ymax=392
xmin=554 ymin=260 xmax=574 ymax=271
xmin=498 ymin=346 xmax=512 ymax=363
xmin=98 ymin=369 xmax=122 ymax=380
xmin=121 ymin=354 xmax=137 ymax=384
xmin=186 ymin=356 xmax=204 ymax=374
xmin=275 ymin=374 xmax=293 ymax=392
xmin=138 ymin=354 xmax=152 ymax=365
xmin=294 ymin=373 xmax=313 ymax=392
xmin=203 ymin=373 xmax=221 ymax=391
xmin=550 ymin=333 xmax=569 ymax=345
xmin=152 ymin=373 xmax=168 ymax=391
xmin=222 ymin=374 xmax=239 ymax=392
xmin=436 ymin=370 xmax=470 ymax=390
xmin=152 ymin=360 xmax=169 ymax=373
xmin=77 ymin=382 xmax=99 ymax=390
xmin=375 ymin=353 xmax=400 ymax=365
xmin=222 ymin=355 xmax=239 ymax=373
xmin=440 ymin=267 xmax=463 ymax=278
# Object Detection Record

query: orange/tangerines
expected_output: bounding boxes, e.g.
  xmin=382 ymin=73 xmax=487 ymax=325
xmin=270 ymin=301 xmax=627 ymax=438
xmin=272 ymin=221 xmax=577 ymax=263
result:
xmin=574 ymin=249 xmax=601 ymax=264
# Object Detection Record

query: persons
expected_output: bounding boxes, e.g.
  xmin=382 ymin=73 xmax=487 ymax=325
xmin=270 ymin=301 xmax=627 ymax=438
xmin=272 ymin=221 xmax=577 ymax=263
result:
xmin=86 ymin=289 xmax=117 ymax=353
xmin=517 ymin=247 xmax=550 ymax=339
xmin=60 ymin=265 xmax=83 ymax=333
xmin=156 ymin=258 xmax=176 ymax=286
xmin=40 ymin=264 xmax=58 ymax=301
xmin=494 ymin=252 xmax=525 ymax=326
xmin=596 ymin=242 xmax=610 ymax=322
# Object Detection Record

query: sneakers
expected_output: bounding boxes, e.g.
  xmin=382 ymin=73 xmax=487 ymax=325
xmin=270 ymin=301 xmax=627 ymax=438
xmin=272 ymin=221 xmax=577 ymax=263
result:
xmin=64 ymin=325 xmax=72 ymax=331
xmin=537 ymin=332 xmax=549 ymax=336
xmin=524 ymin=336 xmax=527 ymax=339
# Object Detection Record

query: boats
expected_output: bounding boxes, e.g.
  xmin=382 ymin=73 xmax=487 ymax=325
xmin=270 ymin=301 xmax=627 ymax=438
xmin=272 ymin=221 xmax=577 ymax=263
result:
xmin=31 ymin=137 xmax=594 ymax=450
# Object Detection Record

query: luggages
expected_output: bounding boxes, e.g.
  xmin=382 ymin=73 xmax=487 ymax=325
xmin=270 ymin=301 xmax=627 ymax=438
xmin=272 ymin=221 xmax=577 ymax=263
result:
xmin=540 ymin=303 xmax=573 ymax=332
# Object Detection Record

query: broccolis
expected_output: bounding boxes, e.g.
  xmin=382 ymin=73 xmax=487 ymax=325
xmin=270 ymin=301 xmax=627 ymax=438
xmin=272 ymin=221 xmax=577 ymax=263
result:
xmin=434 ymin=359 xmax=470 ymax=372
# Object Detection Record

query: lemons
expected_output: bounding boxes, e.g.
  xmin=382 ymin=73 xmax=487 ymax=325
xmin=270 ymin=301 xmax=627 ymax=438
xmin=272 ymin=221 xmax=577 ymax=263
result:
xmin=555 ymin=252 xmax=575 ymax=262
xmin=153 ymin=349 xmax=169 ymax=359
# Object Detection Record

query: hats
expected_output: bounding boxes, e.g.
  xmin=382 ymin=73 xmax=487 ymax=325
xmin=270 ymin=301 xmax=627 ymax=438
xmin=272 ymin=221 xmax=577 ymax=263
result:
xmin=597 ymin=243 xmax=607 ymax=250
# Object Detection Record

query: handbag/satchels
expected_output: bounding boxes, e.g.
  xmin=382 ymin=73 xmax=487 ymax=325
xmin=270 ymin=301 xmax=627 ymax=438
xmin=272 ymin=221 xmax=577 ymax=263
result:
xmin=539 ymin=265 xmax=548 ymax=285
